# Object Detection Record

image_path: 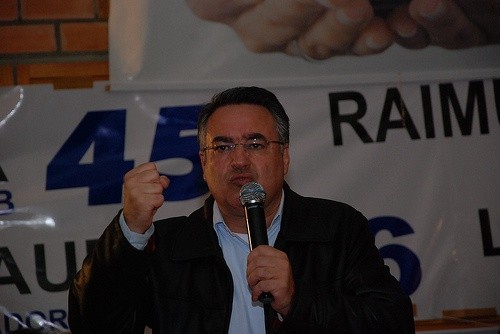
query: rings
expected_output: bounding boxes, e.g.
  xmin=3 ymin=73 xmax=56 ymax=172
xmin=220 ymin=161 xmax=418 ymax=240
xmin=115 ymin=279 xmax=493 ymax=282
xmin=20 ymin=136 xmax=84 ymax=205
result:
xmin=292 ymin=36 xmax=329 ymax=65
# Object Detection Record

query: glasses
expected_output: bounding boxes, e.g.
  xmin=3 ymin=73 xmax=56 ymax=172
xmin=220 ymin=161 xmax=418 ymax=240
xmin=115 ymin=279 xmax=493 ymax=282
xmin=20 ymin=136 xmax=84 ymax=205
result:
xmin=200 ymin=136 xmax=284 ymax=154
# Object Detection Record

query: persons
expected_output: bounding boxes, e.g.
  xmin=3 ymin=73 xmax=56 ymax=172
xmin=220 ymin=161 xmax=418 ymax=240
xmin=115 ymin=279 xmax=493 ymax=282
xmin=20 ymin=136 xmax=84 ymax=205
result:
xmin=189 ymin=0 xmax=500 ymax=65
xmin=69 ymin=87 xmax=417 ymax=334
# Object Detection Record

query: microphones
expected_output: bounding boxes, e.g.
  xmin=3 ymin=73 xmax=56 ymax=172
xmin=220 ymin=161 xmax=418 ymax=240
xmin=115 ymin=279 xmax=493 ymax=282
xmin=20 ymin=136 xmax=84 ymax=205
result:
xmin=239 ymin=182 xmax=275 ymax=303
